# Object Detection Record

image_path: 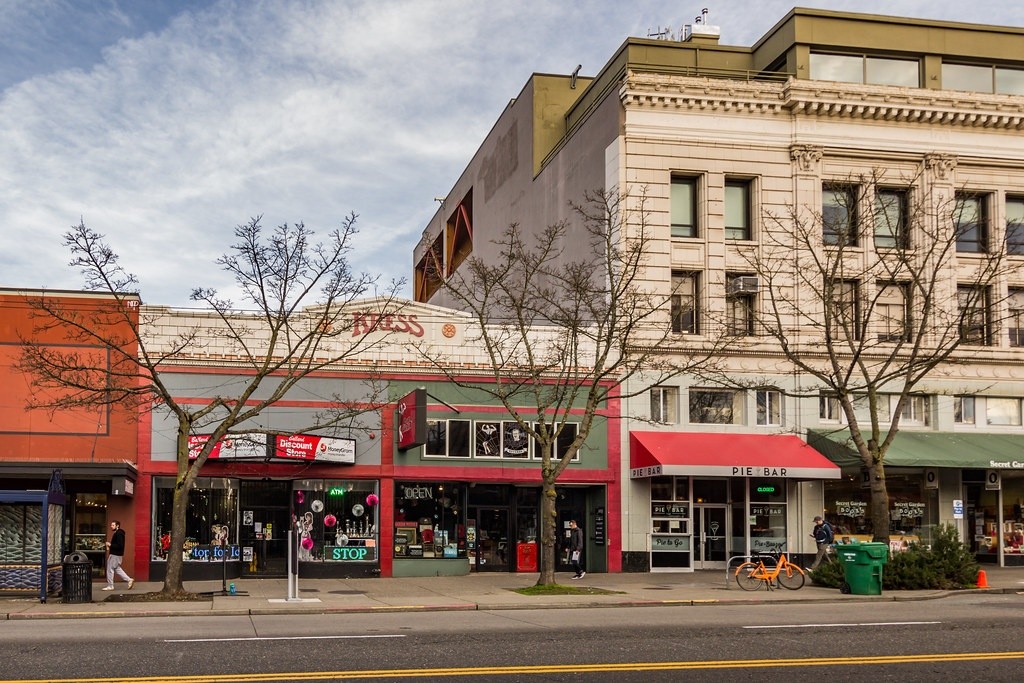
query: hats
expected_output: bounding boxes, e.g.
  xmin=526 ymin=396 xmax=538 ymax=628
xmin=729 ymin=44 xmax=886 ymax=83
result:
xmin=812 ymin=516 xmax=822 ymax=522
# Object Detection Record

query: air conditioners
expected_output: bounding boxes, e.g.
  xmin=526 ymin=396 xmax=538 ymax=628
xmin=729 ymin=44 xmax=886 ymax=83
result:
xmin=729 ymin=278 xmax=759 ymax=295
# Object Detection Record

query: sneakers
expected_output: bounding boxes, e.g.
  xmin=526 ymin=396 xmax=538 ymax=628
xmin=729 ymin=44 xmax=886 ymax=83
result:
xmin=101 ymin=586 xmax=115 ymax=591
xmin=127 ymin=578 xmax=134 ymax=589
xmin=571 ymin=575 xmax=580 ymax=580
xmin=579 ymin=572 xmax=586 ymax=578
xmin=805 ymin=566 xmax=813 ymax=573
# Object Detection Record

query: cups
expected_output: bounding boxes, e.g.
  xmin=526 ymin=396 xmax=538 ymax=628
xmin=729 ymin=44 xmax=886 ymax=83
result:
xmin=230 ymin=583 xmax=234 ymax=591
xmin=231 ymin=586 xmax=235 ymax=594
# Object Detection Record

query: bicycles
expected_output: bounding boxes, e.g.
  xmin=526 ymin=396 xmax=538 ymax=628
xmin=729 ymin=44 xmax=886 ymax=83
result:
xmin=734 ymin=542 xmax=805 ymax=591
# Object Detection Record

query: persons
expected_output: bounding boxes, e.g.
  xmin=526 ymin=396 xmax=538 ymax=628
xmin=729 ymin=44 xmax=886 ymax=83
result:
xmin=566 ymin=520 xmax=586 ymax=580
xmin=101 ymin=521 xmax=134 ymax=591
xmin=806 ymin=516 xmax=833 ymax=574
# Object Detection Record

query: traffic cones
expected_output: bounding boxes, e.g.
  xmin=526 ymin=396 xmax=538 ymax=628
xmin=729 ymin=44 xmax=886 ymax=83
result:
xmin=976 ymin=570 xmax=990 ymax=588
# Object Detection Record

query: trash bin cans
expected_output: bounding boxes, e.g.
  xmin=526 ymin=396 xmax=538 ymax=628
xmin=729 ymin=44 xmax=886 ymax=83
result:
xmin=61 ymin=550 xmax=94 ymax=604
xmin=831 ymin=540 xmax=890 ymax=595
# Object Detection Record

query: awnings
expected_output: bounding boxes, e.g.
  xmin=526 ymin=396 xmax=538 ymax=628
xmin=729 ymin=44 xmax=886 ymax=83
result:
xmin=629 ymin=430 xmax=841 ymax=479
xmin=807 ymin=428 xmax=1024 ymax=471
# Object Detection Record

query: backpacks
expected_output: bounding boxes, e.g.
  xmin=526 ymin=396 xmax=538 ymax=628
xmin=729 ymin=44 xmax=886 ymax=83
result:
xmin=823 ymin=521 xmax=835 ymax=544
